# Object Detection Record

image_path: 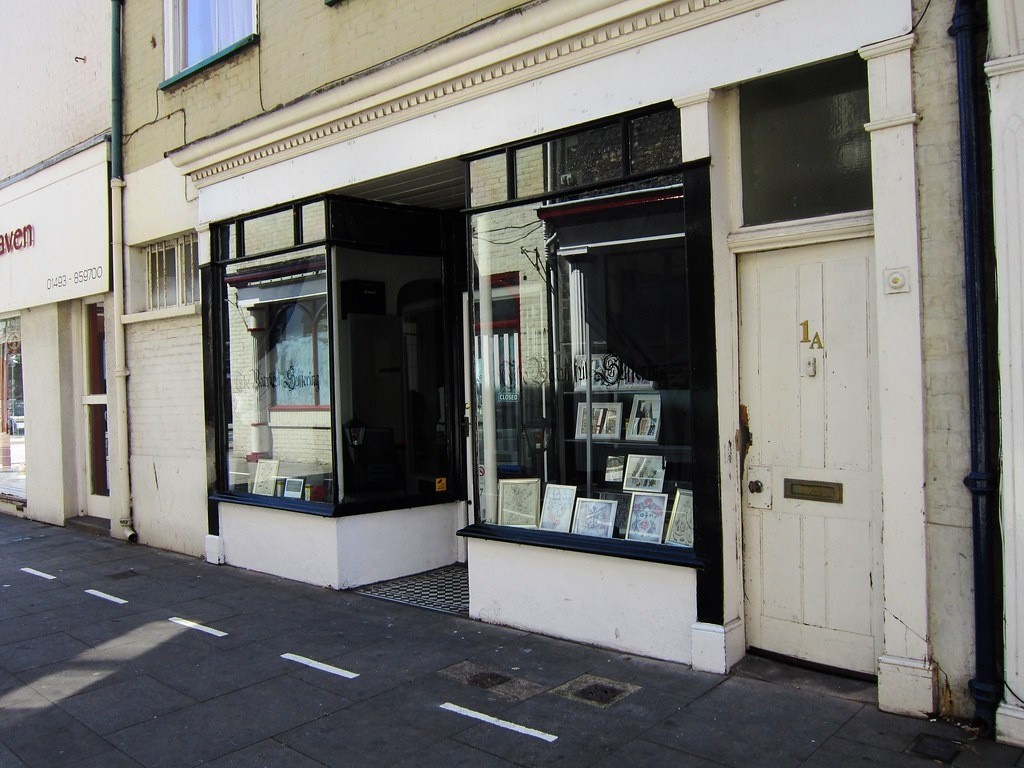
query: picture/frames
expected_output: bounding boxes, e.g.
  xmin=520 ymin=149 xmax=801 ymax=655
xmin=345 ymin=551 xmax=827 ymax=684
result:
xmin=623 ymin=454 xmax=666 ymax=493
xmin=574 ymin=403 xmax=623 ymax=440
xmin=665 ymin=488 xmax=694 ymax=547
xmin=572 ymin=497 xmax=618 ymax=538
xmin=625 ymin=492 xmax=668 ymax=544
xmin=498 ymin=478 xmax=541 ymax=529
xmin=539 ymin=484 xmax=577 ymax=533
xmin=252 ymin=459 xmax=281 ymax=496
xmin=625 ymin=395 xmax=661 ymax=442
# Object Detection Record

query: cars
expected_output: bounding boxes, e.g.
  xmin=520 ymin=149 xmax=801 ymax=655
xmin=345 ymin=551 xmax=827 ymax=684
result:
xmin=10 ymin=396 xmax=25 ymax=434
